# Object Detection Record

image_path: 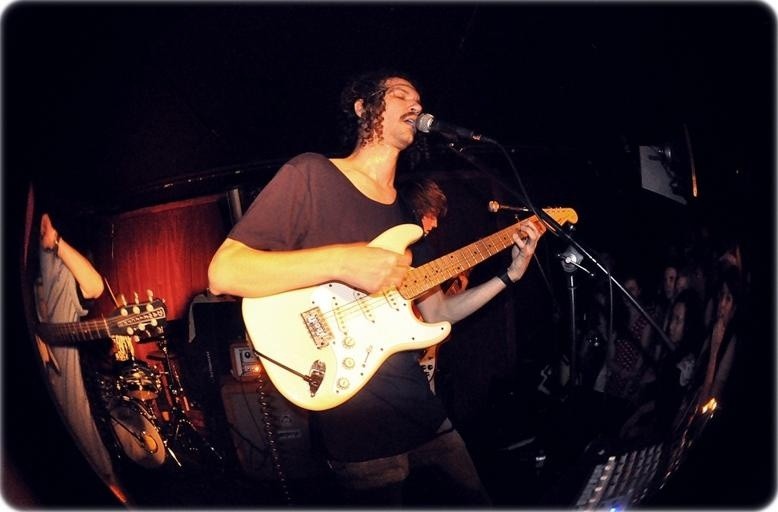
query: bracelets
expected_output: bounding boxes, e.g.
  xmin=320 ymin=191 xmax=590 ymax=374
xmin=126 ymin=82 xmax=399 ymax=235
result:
xmin=52 ymin=233 xmax=61 ymax=257
xmin=498 ymin=269 xmax=514 ymax=287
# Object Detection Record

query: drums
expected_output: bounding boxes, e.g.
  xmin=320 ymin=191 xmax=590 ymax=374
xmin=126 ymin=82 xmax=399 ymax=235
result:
xmin=88 ymin=371 xmax=122 ymax=405
xmin=109 ymin=397 xmax=165 ymax=472
xmin=108 ymin=191 xmax=232 ymax=325
xmin=121 ymin=363 xmax=162 ymax=403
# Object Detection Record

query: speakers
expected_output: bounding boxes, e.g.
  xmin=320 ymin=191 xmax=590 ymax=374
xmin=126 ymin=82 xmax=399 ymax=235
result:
xmin=219 ymin=376 xmax=311 ymax=476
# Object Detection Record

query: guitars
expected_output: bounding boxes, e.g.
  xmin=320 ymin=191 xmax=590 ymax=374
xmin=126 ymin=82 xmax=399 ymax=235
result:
xmin=242 ymin=207 xmax=579 ymax=410
xmin=413 ymin=273 xmax=468 ymax=384
xmin=30 ymin=286 xmax=167 ymax=368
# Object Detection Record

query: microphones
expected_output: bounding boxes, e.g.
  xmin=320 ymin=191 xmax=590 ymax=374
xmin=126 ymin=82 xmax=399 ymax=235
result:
xmin=415 ymin=112 xmax=499 ymax=146
xmin=487 ymin=200 xmax=531 ymax=213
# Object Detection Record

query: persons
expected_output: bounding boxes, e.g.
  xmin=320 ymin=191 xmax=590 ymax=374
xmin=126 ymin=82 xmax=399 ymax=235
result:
xmin=585 ymin=241 xmax=751 ymax=502
xmin=399 ymin=176 xmax=468 ymax=394
xmin=27 ymin=213 xmax=119 ymax=504
xmin=206 ymin=72 xmax=544 ymax=507
